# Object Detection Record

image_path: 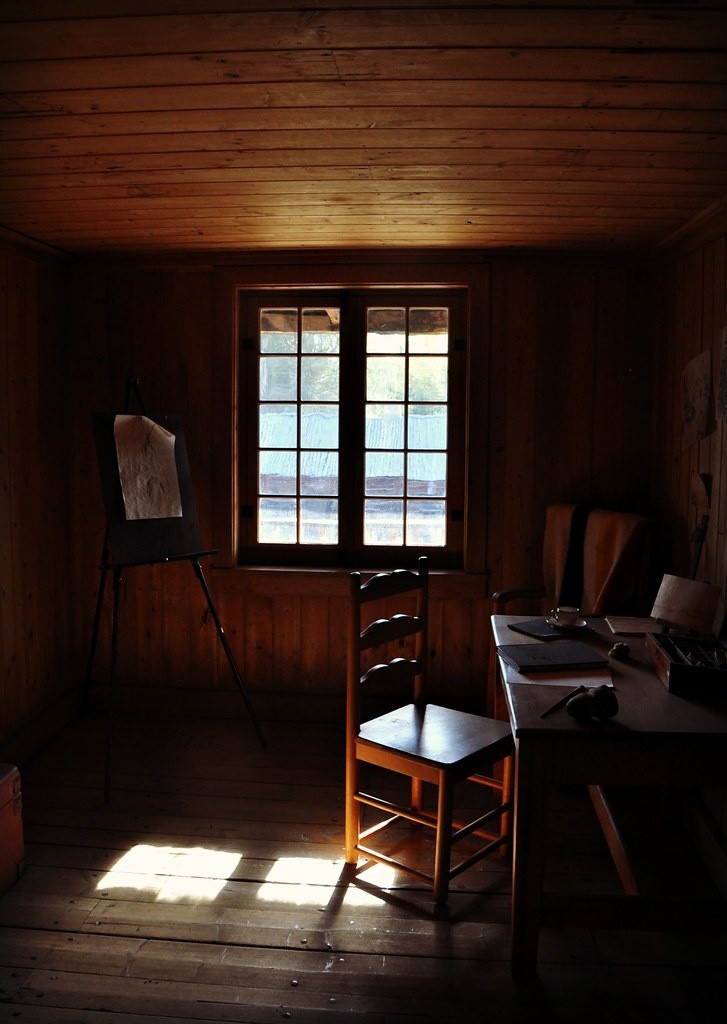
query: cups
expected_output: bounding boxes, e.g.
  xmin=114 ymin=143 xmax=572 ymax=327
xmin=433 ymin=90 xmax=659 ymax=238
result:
xmin=551 ymin=606 xmax=582 ymax=624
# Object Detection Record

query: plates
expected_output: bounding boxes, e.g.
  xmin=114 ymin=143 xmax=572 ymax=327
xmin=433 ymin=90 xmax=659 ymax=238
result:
xmin=546 ymin=617 xmax=588 ymax=630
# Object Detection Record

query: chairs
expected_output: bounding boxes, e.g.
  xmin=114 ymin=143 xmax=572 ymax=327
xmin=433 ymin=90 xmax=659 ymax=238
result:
xmin=488 ymin=501 xmax=651 ymax=720
xmin=344 ymin=556 xmax=516 ymax=906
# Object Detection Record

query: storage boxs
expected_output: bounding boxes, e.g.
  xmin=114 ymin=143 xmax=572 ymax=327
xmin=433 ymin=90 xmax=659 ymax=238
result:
xmin=0 ymin=763 xmax=27 ymax=899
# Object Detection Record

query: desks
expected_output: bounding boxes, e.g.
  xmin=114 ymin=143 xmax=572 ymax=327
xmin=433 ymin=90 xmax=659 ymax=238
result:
xmin=488 ymin=612 xmax=727 ymax=979
